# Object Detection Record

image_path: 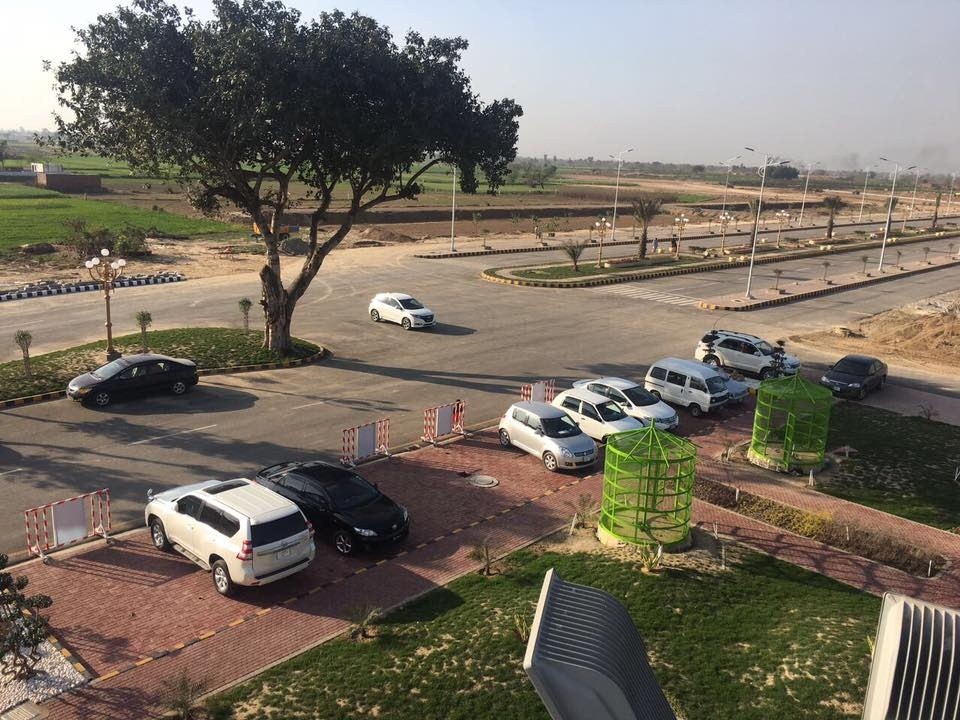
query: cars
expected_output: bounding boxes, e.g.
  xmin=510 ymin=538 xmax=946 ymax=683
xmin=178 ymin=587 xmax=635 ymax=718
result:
xmin=65 ymin=352 xmax=200 ymax=410
xmin=368 ymin=292 xmax=437 ymax=331
xmin=497 ymin=399 xmax=599 ymax=472
xmin=572 ymin=375 xmax=680 ymax=432
xmin=550 ymin=387 xmax=645 ymax=446
xmin=818 ymin=354 xmax=889 ymax=401
xmin=255 ymin=458 xmax=411 ymax=557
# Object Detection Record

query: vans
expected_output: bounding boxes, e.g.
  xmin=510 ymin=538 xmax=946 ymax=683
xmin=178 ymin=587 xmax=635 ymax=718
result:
xmin=643 ymin=357 xmax=731 ymax=419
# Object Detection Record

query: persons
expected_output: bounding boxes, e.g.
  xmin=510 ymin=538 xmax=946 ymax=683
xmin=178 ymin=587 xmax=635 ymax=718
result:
xmin=672 ymin=238 xmax=678 ymax=253
xmin=652 ymin=238 xmax=658 ymax=252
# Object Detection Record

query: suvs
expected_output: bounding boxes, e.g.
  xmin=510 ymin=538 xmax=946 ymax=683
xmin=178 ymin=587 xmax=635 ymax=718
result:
xmin=144 ymin=478 xmax=319 ymax=597
xmin=695 ymin=329 xmax=801 ymax=382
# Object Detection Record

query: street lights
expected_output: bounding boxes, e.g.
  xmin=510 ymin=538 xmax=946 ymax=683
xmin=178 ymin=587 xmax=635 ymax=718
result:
xmin=85 ymin=248 xmax=126 ymax=354
xmin=608 ymin=148 xmax=635 ymax=241
xmin=798 ymin=161 xmax=823 ymax=226
xmin=595 ymin=218 xmax=612 ymax=269
xmin=858 ymin=165 xmax=879 ymax=222
xmin=775 ymin=210 xmax=790 ymax=249
xmin=745 ymin=153 xmax=792 ymax=299
xmin=720 ymin=212 xmax=734 ymax=255
xmin=877 ymin=157 xmax=918 ymax=271
xmin=719 ymin=145 xmax=755 ymax=232
xmin=675 ymin=213 xmax=690 ymax=261
